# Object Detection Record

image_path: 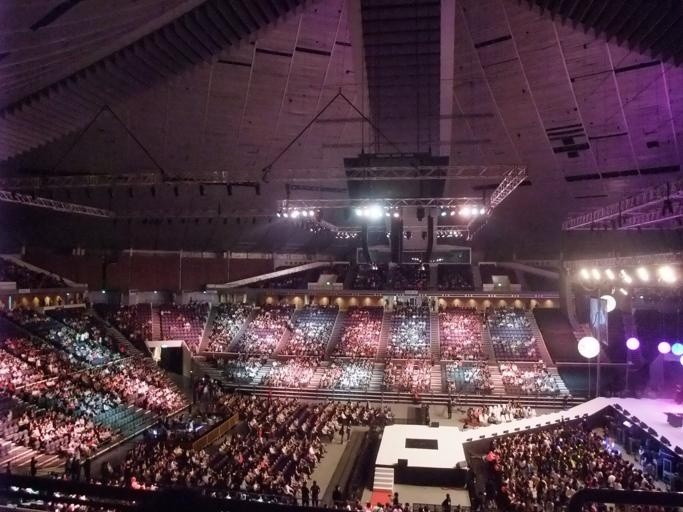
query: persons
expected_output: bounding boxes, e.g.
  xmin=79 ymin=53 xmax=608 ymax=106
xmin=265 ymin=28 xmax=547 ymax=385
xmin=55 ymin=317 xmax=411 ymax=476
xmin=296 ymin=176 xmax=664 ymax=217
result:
xmin=0 ymin=254 xmax=683 ymax=512
